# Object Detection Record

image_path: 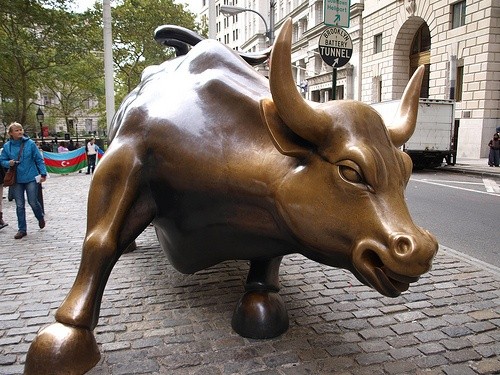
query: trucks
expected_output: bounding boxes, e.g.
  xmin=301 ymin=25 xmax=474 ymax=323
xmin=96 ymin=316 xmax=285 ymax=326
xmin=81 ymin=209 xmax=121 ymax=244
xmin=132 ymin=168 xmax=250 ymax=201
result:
xmin=369 ymin=98 xmax=455 ymax=173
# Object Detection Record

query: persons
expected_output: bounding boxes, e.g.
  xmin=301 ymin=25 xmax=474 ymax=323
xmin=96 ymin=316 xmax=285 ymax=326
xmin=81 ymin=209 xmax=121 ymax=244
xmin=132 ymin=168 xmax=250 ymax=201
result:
xmin=84 ymin=137 xmax=97 ymax=174
xmin=487 ymin=127 xmax=500 ymax=167
xmin=31 ymin=138 xmax=84 ymax=175
xmin=0 ymin=122 xmax=47 ymax=240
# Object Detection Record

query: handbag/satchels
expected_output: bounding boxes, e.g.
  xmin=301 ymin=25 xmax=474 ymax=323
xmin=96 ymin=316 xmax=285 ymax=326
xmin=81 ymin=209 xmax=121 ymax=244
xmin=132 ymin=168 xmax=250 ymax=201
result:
xmin=3 ymin=164 xmax=17 ymax=186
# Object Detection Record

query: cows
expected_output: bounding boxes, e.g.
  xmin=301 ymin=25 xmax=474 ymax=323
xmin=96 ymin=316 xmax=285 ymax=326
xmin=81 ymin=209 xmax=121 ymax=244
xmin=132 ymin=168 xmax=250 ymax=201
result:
xmin=22 ymin=13 xmax=440 ymax=375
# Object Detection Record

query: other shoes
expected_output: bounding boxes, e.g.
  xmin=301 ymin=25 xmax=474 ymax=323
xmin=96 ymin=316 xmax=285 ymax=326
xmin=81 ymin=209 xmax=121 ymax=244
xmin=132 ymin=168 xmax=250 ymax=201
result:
xmin=86 ymin=172 xmax=90 ymax=174
xmin=16 ymin=231 xmax=26 ymax=239
xmin=0 ymin=223 xmax=8 ymax=229
xmin=39 ymin=218 xmax=45 ymax=229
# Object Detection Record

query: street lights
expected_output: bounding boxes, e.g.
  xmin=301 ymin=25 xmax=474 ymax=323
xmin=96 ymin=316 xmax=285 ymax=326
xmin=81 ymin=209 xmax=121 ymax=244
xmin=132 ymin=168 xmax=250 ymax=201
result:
xmin=36 ymin=108 xmax=45 ymax=143
xmin=220 ymin=0 xmax=275 ymax=47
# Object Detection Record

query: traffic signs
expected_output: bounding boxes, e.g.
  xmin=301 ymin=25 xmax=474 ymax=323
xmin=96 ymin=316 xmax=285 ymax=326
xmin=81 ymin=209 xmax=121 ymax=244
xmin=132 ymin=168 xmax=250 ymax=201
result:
xmin=319 ymin=26 xmax=353 ymax=68
xmin=322 ymin=0 xmax=351 ymax=29
